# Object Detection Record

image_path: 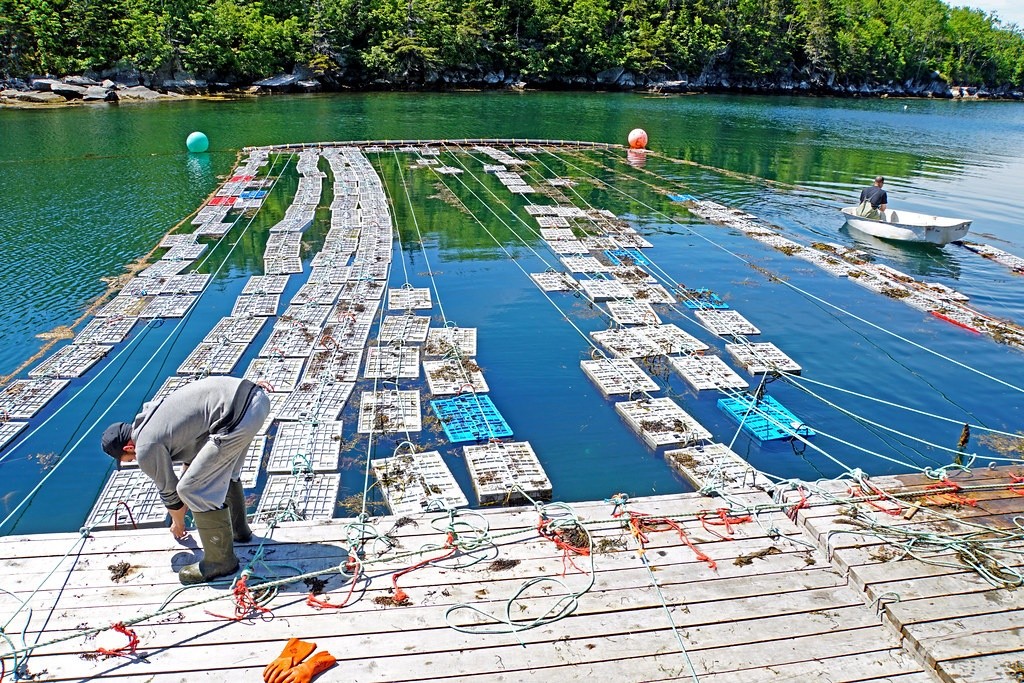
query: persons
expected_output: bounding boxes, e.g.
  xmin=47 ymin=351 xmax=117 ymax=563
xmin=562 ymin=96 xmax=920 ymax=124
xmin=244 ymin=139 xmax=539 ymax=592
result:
xmin=100 ymin=377 xmax=270 ymax=582
xmin=860 ymin=175 xmax=887 ymax=220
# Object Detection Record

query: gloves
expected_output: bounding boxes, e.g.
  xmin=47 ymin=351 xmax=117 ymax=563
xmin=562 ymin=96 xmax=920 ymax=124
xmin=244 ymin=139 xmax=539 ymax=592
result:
xmin=263 ymin=638 xmax=337 ymax=683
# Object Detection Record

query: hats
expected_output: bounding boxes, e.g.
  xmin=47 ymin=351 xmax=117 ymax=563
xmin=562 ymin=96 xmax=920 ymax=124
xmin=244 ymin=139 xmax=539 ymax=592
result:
xmin=102 ymin=422 xmax=132 ymax=471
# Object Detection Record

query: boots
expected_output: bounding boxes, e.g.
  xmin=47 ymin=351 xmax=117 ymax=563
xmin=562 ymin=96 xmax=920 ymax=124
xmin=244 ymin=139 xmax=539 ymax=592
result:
xmin=179 ymin=503 xmax=240 ymax=585
xmin=226 ymin=481 xmax=253 ymax=543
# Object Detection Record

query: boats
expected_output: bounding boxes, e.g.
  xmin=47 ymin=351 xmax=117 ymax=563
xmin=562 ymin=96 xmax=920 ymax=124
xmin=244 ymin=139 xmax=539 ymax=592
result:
xmin=841 ymin=207 xmax=973 ymax=245
